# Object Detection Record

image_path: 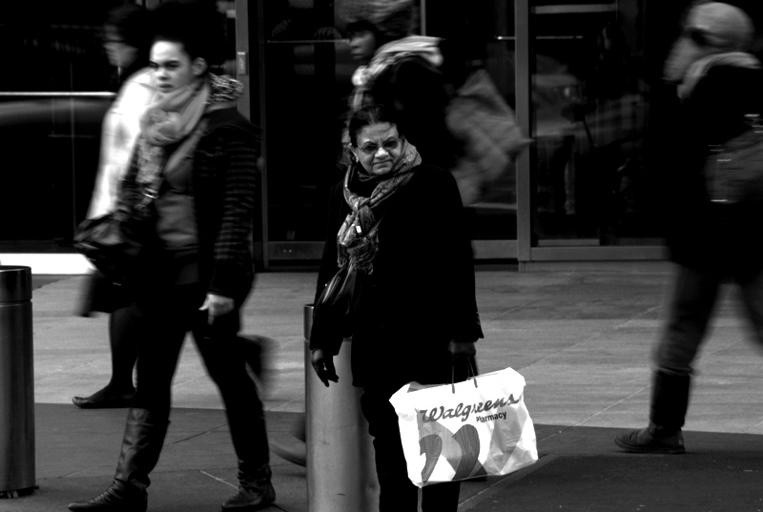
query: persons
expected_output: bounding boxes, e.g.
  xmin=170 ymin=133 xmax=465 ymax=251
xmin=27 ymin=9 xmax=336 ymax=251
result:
xmin=66 ymin=22 xmax=277 ymax=511
xmin=314 ymin=105 xmax=540 ymax=510
xmin=72 ymin=5 xmax=270 ymax=408
xmin=268 ymin=2 xmax=452 ymax=466
xmin=613 ymin=1 xmax=762 ymax=454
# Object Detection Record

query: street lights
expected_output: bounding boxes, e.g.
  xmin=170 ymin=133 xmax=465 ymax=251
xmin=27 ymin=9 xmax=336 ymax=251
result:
xmin=437 ymin=70 xmax=535 ymax=209
xmin=74 ymin=196 xmax=164 ymax=289
xmin=689 ymin=122 xmax=762 ymax=267
xmin=389 ymin=353 xmax=538 ymax=488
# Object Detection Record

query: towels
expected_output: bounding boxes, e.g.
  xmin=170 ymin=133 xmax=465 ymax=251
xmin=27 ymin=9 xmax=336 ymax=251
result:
xmin=0 ymin=265 xmax=34 ymax=498
xmin=303 ymin=304 xmax=380 ymax=512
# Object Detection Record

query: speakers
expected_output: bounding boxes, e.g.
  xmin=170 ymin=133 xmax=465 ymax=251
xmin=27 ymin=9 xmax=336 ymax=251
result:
xmin=68 ymin=409 xmax=171 ymax=511
xmin=220 ymin=405 xmax=276 ymax=512
xmin=614 ymin=373 xmax=689 ymax=454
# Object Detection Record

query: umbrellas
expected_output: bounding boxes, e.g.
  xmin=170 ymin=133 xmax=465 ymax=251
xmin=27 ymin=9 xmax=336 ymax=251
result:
xmin=72 ymin=389 xmax=138 ymax=409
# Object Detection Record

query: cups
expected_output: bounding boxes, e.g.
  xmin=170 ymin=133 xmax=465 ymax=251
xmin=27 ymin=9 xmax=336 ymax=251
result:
xmin=361 ymin=137 xmax=399 ymax=154
